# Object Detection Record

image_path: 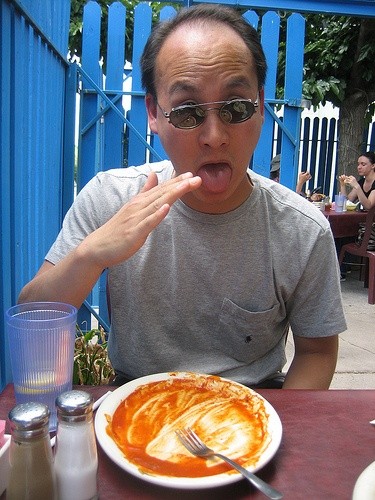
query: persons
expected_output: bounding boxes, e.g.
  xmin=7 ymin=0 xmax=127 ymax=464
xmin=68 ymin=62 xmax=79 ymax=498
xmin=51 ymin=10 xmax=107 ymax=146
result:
xmin=337 ymin=152 xmax=375 ymax=281
xmin=17 ymin=4 xmax=349 ymax=391
xmin=270 ymin=154 xmax=322 ymax=202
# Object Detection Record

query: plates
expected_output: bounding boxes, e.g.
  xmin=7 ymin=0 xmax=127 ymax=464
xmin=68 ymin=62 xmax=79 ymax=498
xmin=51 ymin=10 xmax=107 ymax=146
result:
xmin=94 ymin=372 xmax=282 ymax=490
xmin=352 ymin=460 xmax=375 ymax=500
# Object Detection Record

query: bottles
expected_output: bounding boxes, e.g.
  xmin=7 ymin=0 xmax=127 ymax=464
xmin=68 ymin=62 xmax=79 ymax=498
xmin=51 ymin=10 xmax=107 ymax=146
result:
xmin=8 ymin=402 xmax=56 ymax=500
xmin=54 ymin=390 xmax=100 ymax=500
xmin=321 ymin=196 xmax=331 ymax=212
xmin=305 ymin=190 xmax=311 ymax=201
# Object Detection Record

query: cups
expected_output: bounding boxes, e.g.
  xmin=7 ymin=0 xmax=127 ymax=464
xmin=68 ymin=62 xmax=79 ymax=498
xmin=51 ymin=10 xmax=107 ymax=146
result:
xmin=4 ymin=303 xmax=77 ymax=432
xmin=335 ymin=195 xmax=345 ymax=212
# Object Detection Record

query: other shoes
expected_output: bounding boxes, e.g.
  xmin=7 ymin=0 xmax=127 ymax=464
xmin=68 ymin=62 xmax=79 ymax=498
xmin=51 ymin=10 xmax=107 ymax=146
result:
xmin=344 ymin=268 xmax=352 ymax=274
xmin=339 ymin=273 xmax=346 ymax=282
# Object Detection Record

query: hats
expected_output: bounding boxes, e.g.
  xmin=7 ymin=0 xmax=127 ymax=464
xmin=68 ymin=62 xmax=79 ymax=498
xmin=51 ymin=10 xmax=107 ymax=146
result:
xmin=270 ymin=153 xmax=283 ymax=175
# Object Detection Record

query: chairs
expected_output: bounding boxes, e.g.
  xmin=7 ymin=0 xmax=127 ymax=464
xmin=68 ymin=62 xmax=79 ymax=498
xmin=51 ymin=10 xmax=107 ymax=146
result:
xmin=338 ymin=204 xmax=375 ymax=305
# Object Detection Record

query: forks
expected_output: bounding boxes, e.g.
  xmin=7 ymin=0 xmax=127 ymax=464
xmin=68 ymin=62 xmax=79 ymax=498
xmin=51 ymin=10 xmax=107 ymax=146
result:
xmin=174 ymin=426 xmax=286 ymax=500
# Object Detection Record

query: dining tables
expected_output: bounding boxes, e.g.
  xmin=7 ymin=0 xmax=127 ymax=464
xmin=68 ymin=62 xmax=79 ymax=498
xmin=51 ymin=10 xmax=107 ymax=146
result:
xmin=1 ymin=382 xmax=375 ymax=500
xmin=301 ymin=193 xmax=368 ymax=281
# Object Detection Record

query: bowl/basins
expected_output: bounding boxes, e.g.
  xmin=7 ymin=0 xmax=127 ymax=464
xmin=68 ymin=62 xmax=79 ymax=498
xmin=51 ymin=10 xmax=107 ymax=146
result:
xmin=346 ymin=205 xmax=356 ymax=211
xmin=310 ymin=201 xmax=322 ymax=208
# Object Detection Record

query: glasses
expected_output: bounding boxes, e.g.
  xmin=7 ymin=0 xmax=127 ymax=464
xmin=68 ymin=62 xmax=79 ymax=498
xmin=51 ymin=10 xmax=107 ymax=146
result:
xmin=155 ymin=91 xmax=260 ymax=131
